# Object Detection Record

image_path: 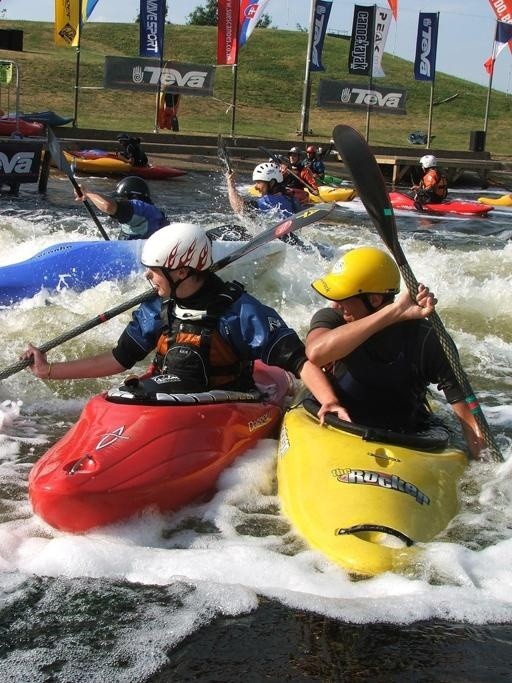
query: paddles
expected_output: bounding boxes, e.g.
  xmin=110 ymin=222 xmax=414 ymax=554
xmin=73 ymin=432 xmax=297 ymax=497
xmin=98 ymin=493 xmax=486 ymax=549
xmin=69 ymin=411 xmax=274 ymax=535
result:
xmin=257 ymin=146 xmax=325 ymax=203
xmin=333 ymin=122 xmax=504 ymax=465
xmin=0 ymin=200 xmax=338 ymax=382
xmin=410 ymin=171 xmax=419 ymax=202
xmin=170 ymin=94 xmax=180 ymax=133
xmin=217 ymin=134 xmax=236 ymax=177
xmin=47 ymin=128 xmax=112 ymax=243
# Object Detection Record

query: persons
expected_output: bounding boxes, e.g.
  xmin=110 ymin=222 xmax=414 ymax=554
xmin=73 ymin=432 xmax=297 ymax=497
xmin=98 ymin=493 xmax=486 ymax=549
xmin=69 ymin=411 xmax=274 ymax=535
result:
xmin=302 ymin=245 xmax=494 ymax=464
xmin=222 ymin=143 xmax=449 ymax=230
xmin=115 ymin=132 xmax=149 ymax=169
xmin=73 ymin=175 xmax=171 ymax=246
xmin=16 ymin=221 xmax=352 ymax=430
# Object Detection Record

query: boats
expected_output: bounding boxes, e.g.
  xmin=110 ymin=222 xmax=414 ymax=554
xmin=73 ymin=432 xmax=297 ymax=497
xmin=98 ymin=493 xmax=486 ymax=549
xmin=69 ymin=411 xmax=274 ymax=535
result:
xmin=39 ymin=147 xmax=188 ymax=179
xmin=7 ymin=109 xmax=73 ymax=128
xmin=26 ymin=360 xmax=293 ymax=544
xmin=1 ymin=115 xmax=45 ymax=136
xmin=246 ymin=185 xmax=356 ymax=204
xmin=313 ymin=173 xmax=343 ymax=187
xmin=478 ymin=191 xmax=512 ymax=206
xmin=0 ymin=229 xmax=290 ymax=326
xmin=387 ymin=192 xmax=494 ymax=216
xmin=156 ymin=58 xmax=181 ymax=131
xmin=31 ymin=374 xmax=287 ymax=532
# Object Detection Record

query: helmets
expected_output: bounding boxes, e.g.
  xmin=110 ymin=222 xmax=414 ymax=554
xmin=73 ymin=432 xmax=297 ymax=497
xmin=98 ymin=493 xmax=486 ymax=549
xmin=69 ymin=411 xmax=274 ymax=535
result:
xmin=116 ymin=176 xmax=149 ymax=198
xmin=141 ymin=224 xmax=212 ymax=272
xmin=290 ymin=146 xmax=316 ymax=154
xmin=253 ymin=163 xmax=283 ymax=183
xmin=311 ymin=247 xmax=400 ymax=301
xmin=419 ymin=155 xmax=437 ymax=168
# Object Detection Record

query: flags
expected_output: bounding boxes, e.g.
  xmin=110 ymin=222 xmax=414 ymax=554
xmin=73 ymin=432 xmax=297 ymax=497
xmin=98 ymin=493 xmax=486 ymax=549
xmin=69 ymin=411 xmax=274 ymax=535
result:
xmin=413 ymin=11 xmax=439 ymax=82
xmin=372 ymin=7 xmax=394 ymax=78
xmin=483 ymin=21 xmax=512 ymax=75
xmin=215 ymin=0 xmax=241 ymax=66
xmin=139 ymin=0 xmax=164 ymax=57
xmin=54 ymin=0 xmax=80 ymax=50
xmin=348 ymin=4 xmax=377 ymax=77
xmin=239 ymin=0 xmax=271 ymax=49
xmin=309 ymin=0 xmax=333 ymax=71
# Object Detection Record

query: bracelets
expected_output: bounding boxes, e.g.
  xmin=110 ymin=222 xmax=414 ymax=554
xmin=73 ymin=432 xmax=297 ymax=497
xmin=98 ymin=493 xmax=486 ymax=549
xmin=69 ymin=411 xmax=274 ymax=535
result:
xmin=47 ymin=361 xmax=56 ymax=382
